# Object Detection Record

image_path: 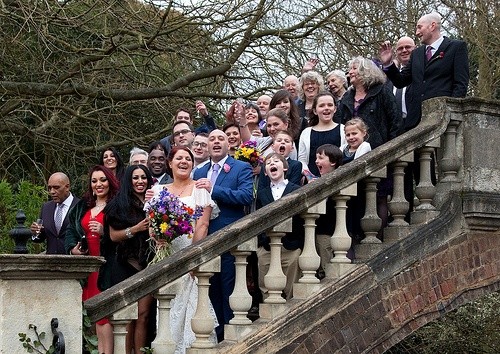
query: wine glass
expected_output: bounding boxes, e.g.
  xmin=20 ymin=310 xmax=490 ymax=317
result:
xmin=89 ymin=214 xmax=98 ymax=238
xmin=33 ymin=218 xmax=44 ymax=239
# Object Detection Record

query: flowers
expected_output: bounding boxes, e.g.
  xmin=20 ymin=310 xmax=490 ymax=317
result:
xmin=435 ymin=52 xmax=445 ymax=58
xmin=142 ymin=187 xmax=204 ymax=272
xmin=234 ymin=141 xmax=267 ymax=198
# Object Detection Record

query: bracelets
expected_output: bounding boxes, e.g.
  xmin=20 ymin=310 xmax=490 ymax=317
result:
xmin=125 ymin=228 xmax=134 ymax=238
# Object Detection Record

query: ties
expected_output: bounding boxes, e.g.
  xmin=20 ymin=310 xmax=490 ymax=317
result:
xmin=190 ymin=167 xmax=198 ymax=174
xmin=152 ymin=178 xmax=158 ymax=184
xmin=395 ymin=67 xmax=407 ymax=116
xmin=54 ymin=204 xmax=64 ymax=235
xmin=426 ymin=45 xmax=434 ymax=60
xmin=210 ymin=164 xmax=221 ymax=186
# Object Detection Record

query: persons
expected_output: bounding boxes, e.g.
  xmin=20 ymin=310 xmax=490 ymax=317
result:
xmin=130 ymin=148 xmax=149 ymax=169
xmin=385 ymin=59 xmax=400 ymax=96
xmin=103 ymin=164 xmax=154 ymax=354
xmin=63 ymin=166 xmax=120 ymax=354
xmin=146 ymin=149 xmax=173 ymax=185
xmin=377 ymin=12 xmax=469 ymax=185
xmin=256 ymin=152 xmax=304 ymax=303
xmin=31 ymin=172 xmax=80 ymax=254
xmin=305 ymin=143 xmax=344 ymax=279
xmin=158 ymin=56 xmax=350 ymax=219
xmin=338 ymin=118 xmax=371 ymax=263
xmin=146 ymin=129 xmax=254 ymax=344
xmin=338 ymin=55 xmax=403 ymax=242
xmin=386 ymin=35 xmax=417 ymax=130
xmin=143 ymin=148 xmax=220 ymax=354
xmin=99 ymin=146 xmax=125 ymax=182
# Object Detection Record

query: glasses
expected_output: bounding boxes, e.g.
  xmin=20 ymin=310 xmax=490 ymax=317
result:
xmin=397 ymin=45 xmax=415 ymax=52
xmin=172 ymin=130 xmax=193 ymax=138
